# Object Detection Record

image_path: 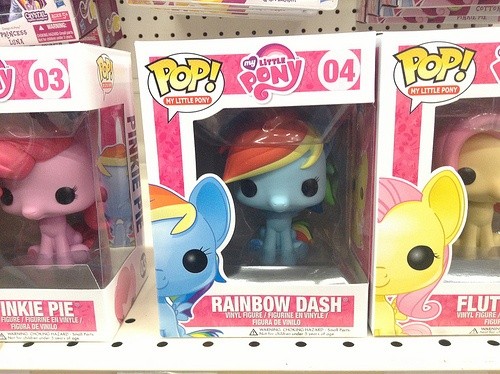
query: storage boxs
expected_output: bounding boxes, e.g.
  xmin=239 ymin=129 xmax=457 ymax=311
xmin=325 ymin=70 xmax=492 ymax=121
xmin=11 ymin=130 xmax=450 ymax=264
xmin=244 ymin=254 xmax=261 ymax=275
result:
xmin=367 ymin=25 xmax=500 ymax=339
xmin=134 ymin=30 xmax=377 ymax=342
xmin=0 ymin=42 xmax=149 ymax=347
